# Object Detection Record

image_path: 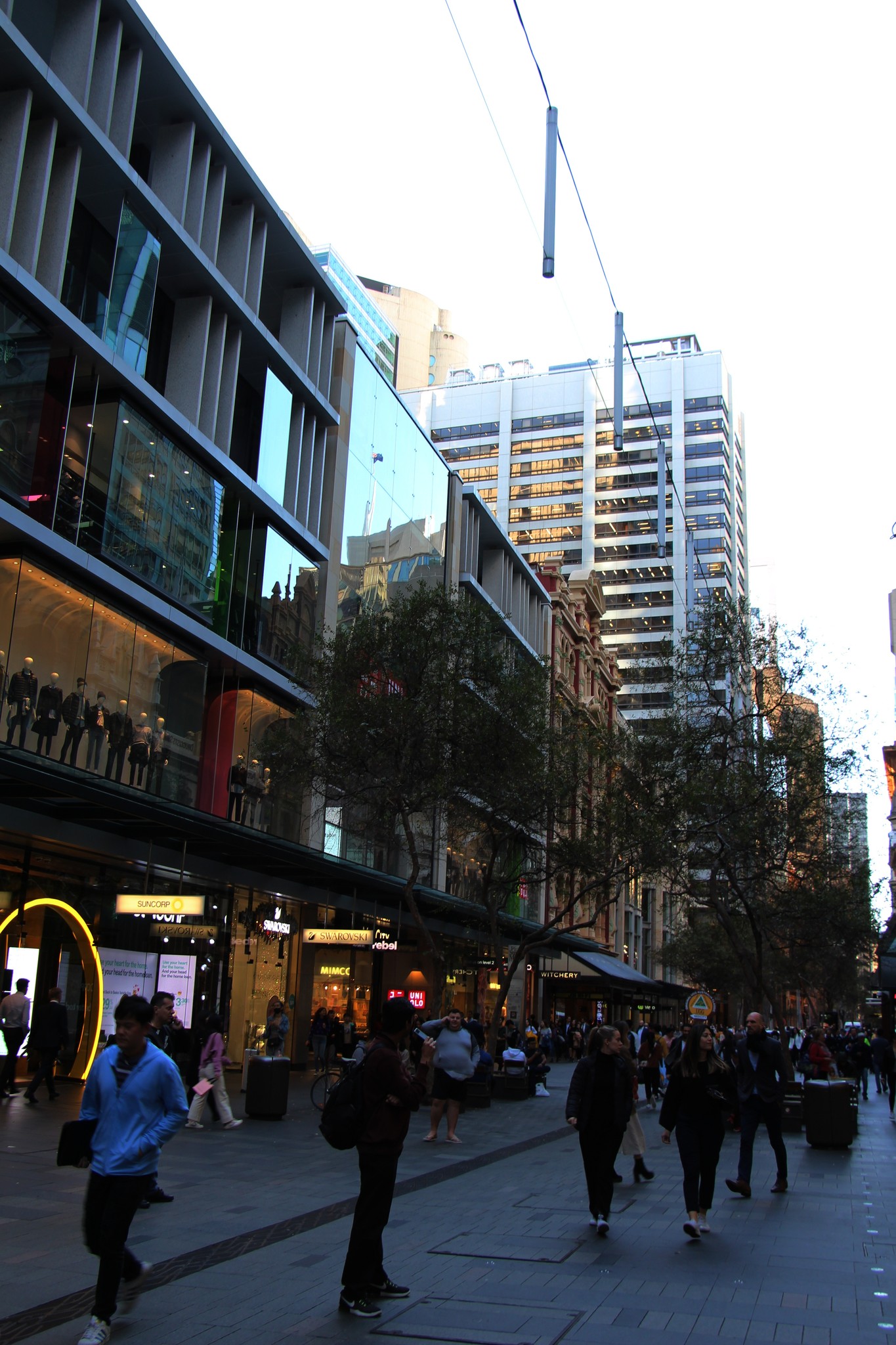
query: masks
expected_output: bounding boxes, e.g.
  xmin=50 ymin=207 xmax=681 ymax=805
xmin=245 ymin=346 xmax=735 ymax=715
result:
xmin=274 ymin=1006 xmax=283 ymax=1014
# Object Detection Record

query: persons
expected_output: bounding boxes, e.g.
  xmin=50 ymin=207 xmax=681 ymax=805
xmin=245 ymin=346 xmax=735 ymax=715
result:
xmin=623 ymin=1021 xmax=748 ymax=1132
xmin=727 ymin=1011 xmax=787 ymax=1196
xmin=75 ymin=994 xmax=189 ymax=1345
xmin=308 ymin=1005 xmax=444 ymax=1080
xmin=186 ymin=1010 xmax=243 ymax=1129
xmin=0 ymin=979 xmax=30 ymax=1098
xmin=458 ymin=1010 xmax=603 ymax=1097
xmin=137 ymin=991 xmax=193 ymax=1208
xmin=764 ymin=1022 xmax=896 ymax=1119
xmin=564 ymin=1022 xmax=633 ymax=1236
xmin=24 ymin=987 xmax=69 ymax=1103
xmin=422 ymin=1008 xmax=481 ymax=1143
xmin=658 ymin=1023 xmax=732 ymax=1238
xmin=610 ymin=1020 xmax=654 ymax=1182
xmin=338 ymin=997 xmax=437 ymax=1319
xmin=258 ymin=1000 xmax=290 ymax=1056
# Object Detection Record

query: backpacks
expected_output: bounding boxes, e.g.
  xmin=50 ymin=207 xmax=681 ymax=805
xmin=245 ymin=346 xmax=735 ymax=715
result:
xmin=318 ymin=1047 xmax=389 ymax=1151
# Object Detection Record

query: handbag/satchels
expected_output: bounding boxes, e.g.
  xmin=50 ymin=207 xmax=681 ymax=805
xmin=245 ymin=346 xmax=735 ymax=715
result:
xmin=795 ymin=1052 xmax=819 ymax=1077
xmin=267 ymin=1037 xmax=280 ymax=1047
xmin=191 ymin=1076 xmax=218 ymax=1097
xmin=535 ymin=1081 xmax=550 ymax=1097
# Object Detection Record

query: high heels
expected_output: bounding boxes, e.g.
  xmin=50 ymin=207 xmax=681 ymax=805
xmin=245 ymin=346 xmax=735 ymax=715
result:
xmin=631 ymin=1159 xmax=655 ymax=1181
xmin=613 ymin=1169 xmax=624 ymax=1182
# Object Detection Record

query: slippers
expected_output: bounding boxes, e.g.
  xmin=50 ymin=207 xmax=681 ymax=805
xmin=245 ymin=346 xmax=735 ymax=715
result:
xmin=444 ymin=1137 xmax=461 ymax=1143
xmin=423 ymin=1135 xmax=437 ymax=1142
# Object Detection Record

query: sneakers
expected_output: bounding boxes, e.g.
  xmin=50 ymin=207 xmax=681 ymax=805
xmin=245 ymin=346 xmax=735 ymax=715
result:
xmin=337 ymin=1292 xmax=382 ymax=1319
xmin=683 ymin=1219 xmax=702 ymax=1237
xmin=697 ymin=1214 xmax=711 ymax=1231
xmin=368 ymin=1276 xmax=412 ymax=1298
xmin=590 ymin=1215 xmax=596 ymax=1225
xmin=77 ymin=1314 xmax=111 ymax=1345
xmin=121 ymin=1260 xmax=151 ymax=1300
xmin=596 ymin=1218 xmax=611 ymax=1236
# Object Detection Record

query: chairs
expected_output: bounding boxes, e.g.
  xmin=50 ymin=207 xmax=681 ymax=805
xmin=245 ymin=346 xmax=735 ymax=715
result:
xmin=466 ymin=1056 xmax=546 ymax=1107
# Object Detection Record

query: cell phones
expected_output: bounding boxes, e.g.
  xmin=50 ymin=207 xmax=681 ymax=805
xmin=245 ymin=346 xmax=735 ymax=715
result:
xmin=446 ymin=1020 xmax=449 ymax=1027
xmin=414 ymin=1028 xmax=434 ymax=1043
xmin=167 ymin=1018 xmax=173 ymax=1024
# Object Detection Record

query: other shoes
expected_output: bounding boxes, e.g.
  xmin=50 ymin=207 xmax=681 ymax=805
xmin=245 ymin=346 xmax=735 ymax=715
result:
xmin=313 ymin=1073 xmax=318 ymax=1076
xmin=186 ymin=1120 xmax=203 ymax=1128
xmin=9 ymin=1088 xmax=21 ymax=1094
xmin=224 ymin=1117 xmax=242 ymax=1129
xmin=321 ymin=1071 xmax=324 ymax=1074
xmin=23 ymin=1090 xmax=39 ymax=1104
xmin=139 ymin=1196 xmax=151 ymax=1209
xmin=769 ymin=1178 xmax=789 ymax=1194
xmin=151 ymin=1188 xmax=174 ymax=1201
xmin=49 ymin=1090 xmax=60 ymax=1101
xmin=0 ymin=1089 xmax=9 ymax=1098
xmin=889 ymin=1111 xmax=895 ymax=1119
xmin=726 ymin=1178 xmax=750 ymax=1196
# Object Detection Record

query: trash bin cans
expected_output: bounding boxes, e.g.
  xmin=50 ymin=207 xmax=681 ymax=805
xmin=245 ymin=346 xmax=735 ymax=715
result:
xmin=245 ymin=1055 xmax=290 ymax=1121
xmin=805 ymin=1079 xmax=859 ymax=1151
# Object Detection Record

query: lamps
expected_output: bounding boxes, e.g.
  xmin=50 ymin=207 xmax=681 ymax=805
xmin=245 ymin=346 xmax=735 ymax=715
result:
xmin=323 ymin=983 xmax=369 ymax=992
xmin=201 ymin=995 xmax=208 ymax=1000
xmin=412 ymin=961 xmax=421 ymax=970
xmin=191 ymin=937 xmax=196 ymax=944
xmin=209 ymin=938 xmax=216 ymax=944
xmin=212 ymin=903 xmax=219 ymax=909
xmin=163 ymin=935 xmax=169 ymax=943
xmin=207 ymin=956 xmax=213 ymax=963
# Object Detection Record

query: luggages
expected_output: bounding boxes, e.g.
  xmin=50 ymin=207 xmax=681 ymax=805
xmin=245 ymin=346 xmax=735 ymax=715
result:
xmin=241 ymin=1036 xmax=261 ymax=1092
